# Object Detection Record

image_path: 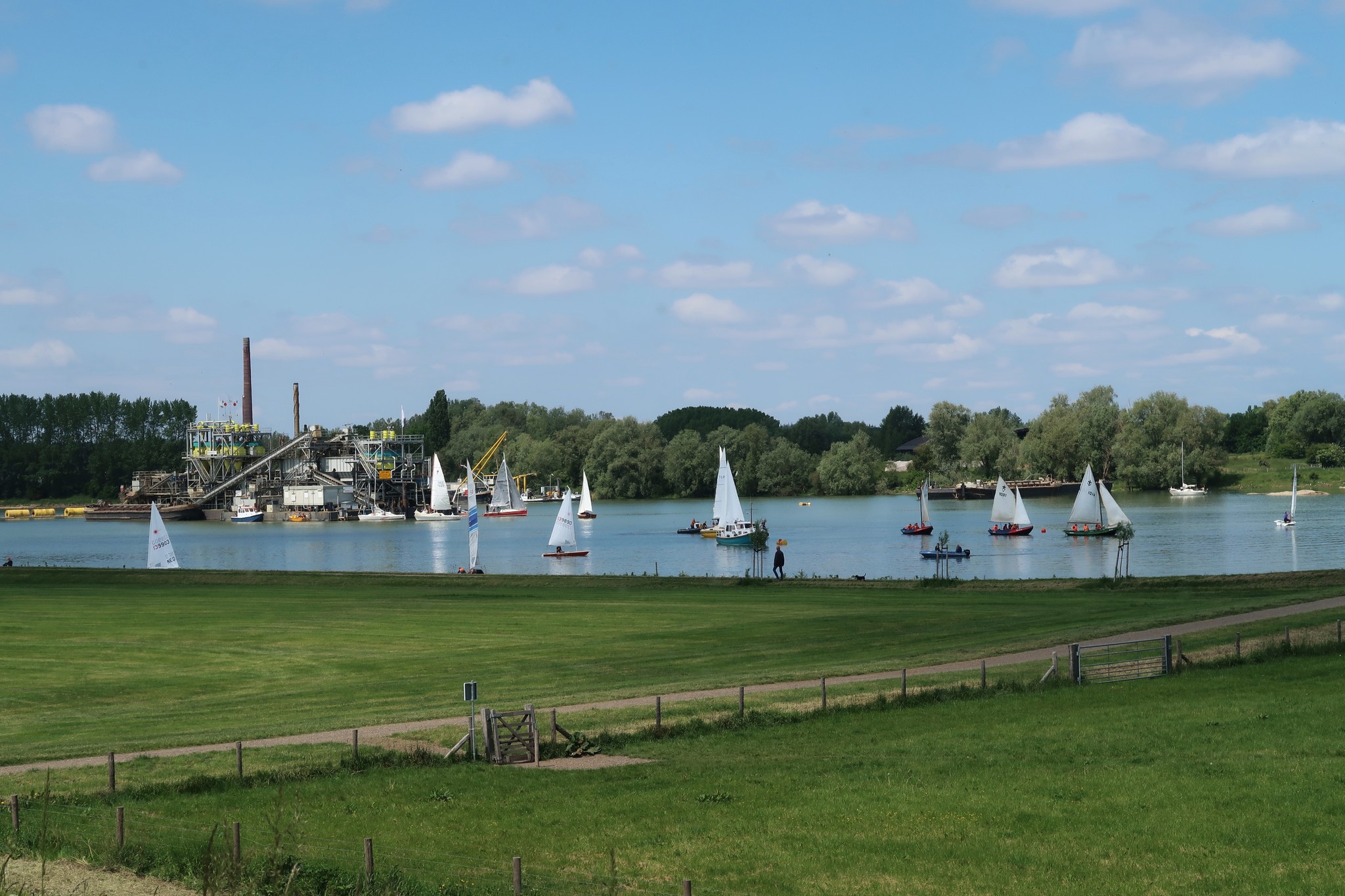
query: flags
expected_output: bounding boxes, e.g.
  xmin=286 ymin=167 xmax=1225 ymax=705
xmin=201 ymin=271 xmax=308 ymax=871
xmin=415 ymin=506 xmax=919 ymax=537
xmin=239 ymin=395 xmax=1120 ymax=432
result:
xmin=219 ymin=399 xmax=224 ymax=408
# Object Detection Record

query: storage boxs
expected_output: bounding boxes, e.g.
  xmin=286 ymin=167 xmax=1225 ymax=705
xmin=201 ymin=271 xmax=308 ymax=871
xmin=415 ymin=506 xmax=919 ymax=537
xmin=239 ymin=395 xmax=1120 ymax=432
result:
xmin=132 ymin=480 xmax=151 ymax=492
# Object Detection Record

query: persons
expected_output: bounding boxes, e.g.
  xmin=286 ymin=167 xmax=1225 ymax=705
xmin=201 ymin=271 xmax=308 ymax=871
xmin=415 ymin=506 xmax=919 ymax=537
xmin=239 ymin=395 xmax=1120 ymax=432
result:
xmin=486 ymin=503 xmax=489 ymax=513
xmin=716 ymin=519 xmax=719 ymax=525
xmin=1003 ymin=523 xmax=1018 ymax=530
xmin=993 ymin=525 xmax=1000 ymax=531
xmin=497 ymin=507 xmax=501 ymax=512
xmin=457 ymin=567 xmax=467 ymax=573
xmin=691 ymin=518 xmax=695 ymax=529
xmin=298 ymin=512 xmax=306 ymax=518
xmin=1084 ymin=524 xmax=1090 ymax=531
xmin=1095 ymin=524 xmax=1101 ymax=530
xmin=703 ymin=521 xmax=708 ymax=529
xmin=458 ymin=505 xmax=462 ymax=515
xmin=762 ymin=520 xmax=769 ymax=536
xmin=906 ymin=523 xmax=919 ymax=530
xmin=773 ymin=546 xmax=784 ymax=581
xmin=492 ymin=507 xmax=496 ymax=512
xmin=955 ymin=544 xmax=962 ymax=552
xmin=1284 ymin=511 xmax=1290 ymax=523
xmin=1071 ymin=524 xmax=1077 ymax=531
xmin=935 ymin=543 xmax=943 ymax=552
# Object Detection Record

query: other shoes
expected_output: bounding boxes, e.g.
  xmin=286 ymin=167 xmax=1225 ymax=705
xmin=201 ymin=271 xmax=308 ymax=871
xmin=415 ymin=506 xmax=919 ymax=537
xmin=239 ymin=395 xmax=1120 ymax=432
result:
xmin=777 ymin=578 xmax=779 ymax=580
xmin=781 ymin=579 xmax=783 ymax=581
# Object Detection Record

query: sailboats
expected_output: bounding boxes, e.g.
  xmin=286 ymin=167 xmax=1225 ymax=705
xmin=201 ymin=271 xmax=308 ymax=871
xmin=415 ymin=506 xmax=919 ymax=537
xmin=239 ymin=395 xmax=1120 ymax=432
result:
xmin=542 ymin=488 xmax=590 ymax=557
xmin=576 ymin=468 xmax=597 ymax=519
xmin=1060 ymin=463 xmax=1133 ymax=536
xmin=483 ymin=451 xmax=528 ymax=518
xmin=1274 ymin=467 xmax=1298 ymax=527
xmin=413 ymin=451 xmax=462 ymax=521
xmin=899 ymin=478 xmax=934 ymax=535
xmin=1167 ymin=441 xmax=1209 ymax=496
xmin=987 ymin=475 xmax=1034 ymax=536
xmin=699 ymin=445 xmax=750 ymax=539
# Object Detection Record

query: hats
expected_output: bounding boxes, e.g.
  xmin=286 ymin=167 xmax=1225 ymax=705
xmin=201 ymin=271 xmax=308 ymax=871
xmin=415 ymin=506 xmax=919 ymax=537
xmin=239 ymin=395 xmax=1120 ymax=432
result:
xmin=8 ymin=557 xmax=11 ymax=560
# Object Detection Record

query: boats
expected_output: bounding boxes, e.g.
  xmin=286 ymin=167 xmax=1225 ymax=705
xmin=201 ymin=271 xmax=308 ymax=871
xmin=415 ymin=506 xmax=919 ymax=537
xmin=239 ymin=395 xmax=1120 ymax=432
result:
xmin=676 ymin=521 xmax=704 ymax=534
xmin=715 ymin=518 xmax=769 ymax=546
xmin=288 ymin=511 xmax=308 ymax=522
xmin=357 ymin=503 xmax=406 ymax=520
xmin=230 ymin=503 xmax=264 ymax=523
xmin=520 ymin=485 xmax=581 ymax=503
xmin=445 ymin=473 xmax=498 ymax=504
xmin=83 ymin=498 xmax=202 ymax=521
xmin=918 ymin=549 xmax=970 ymax=559
